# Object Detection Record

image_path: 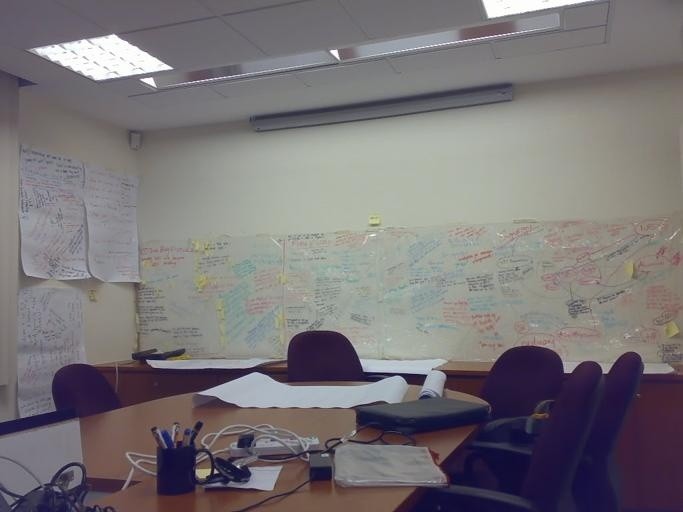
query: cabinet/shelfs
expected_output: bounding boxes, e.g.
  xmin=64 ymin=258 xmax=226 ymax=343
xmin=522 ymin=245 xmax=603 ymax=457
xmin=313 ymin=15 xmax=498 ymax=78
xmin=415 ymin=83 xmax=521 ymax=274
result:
xmin=101 ymin=371 xmax=236 ymax=408
xmin=611 ymin=381 xmax=683 ymax=511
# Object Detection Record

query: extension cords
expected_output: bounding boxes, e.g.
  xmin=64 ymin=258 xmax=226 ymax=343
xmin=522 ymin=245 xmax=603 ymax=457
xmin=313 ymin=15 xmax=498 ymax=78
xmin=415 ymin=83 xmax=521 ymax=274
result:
xmin=224 ymin=435 xmax=320 ymax=458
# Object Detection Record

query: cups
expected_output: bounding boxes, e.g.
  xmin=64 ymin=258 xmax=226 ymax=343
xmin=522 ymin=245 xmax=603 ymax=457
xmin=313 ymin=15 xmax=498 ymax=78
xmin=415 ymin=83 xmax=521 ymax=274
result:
xmin=156 ymin=442 xmax=214 ymax=496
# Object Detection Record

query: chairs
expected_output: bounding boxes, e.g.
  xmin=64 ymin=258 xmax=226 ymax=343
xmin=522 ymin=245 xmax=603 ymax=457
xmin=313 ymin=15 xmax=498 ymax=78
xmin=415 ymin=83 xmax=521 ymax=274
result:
xmin=286 ymin=329 xmax=388 ymax=382
xmin=415 ymin=344 xmax=645 ymax=512
xmin=50 ymin=363 xmax=123 ymax=418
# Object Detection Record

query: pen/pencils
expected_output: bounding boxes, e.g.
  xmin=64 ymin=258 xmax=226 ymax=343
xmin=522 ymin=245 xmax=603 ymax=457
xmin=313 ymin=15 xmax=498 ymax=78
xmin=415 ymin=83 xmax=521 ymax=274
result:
xmin=151 ymin=420 xmax=204 ymax=448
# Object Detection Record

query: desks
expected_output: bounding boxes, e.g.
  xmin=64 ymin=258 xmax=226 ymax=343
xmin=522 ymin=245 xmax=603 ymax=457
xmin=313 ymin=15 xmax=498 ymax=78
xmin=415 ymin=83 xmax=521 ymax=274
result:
xmin=1 ymin=382 xmax=491 ymax=512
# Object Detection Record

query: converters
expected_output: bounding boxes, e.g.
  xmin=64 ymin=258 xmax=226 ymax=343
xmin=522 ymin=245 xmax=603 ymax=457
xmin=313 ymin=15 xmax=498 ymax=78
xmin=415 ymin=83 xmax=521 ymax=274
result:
xmin=309 ymin=452 xmax=332 ymax=480
